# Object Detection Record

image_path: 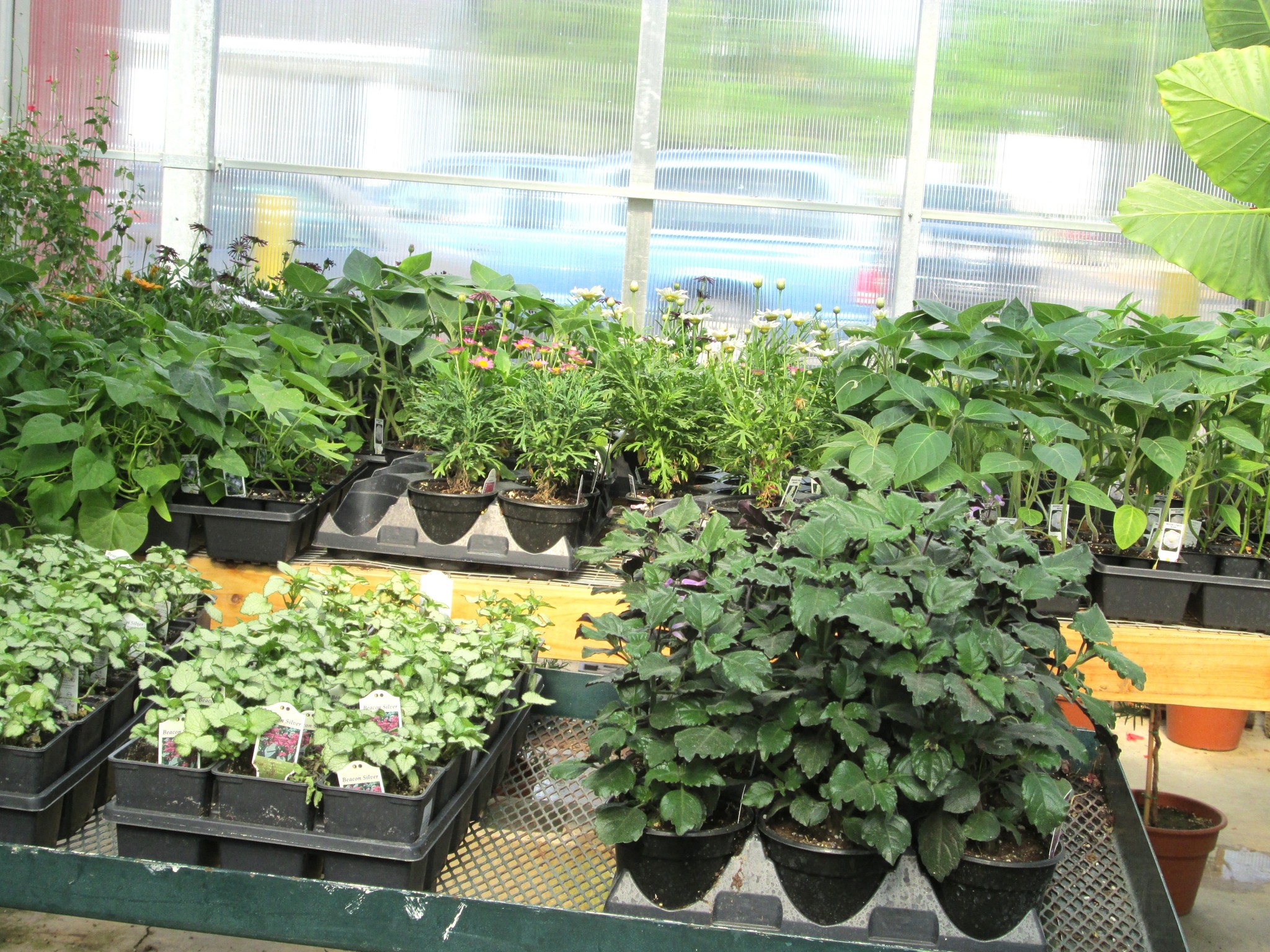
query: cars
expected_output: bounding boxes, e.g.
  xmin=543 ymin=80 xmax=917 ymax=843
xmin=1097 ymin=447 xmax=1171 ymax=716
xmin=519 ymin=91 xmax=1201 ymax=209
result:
xmin=98 ymin=146 xmax=1061 ymax=354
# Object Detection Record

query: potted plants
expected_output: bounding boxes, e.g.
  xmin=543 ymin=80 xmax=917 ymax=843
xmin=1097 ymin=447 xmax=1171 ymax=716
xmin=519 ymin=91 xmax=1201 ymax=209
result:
xmin=574 ymin=471 xmax=1152 ymax=937
xmin=0 ymin=522 xmax=221 ymax=851
xmin=0 ymin=244 xmax=546 ymax=564
xmin=103 ymin=553 xmax=557 ymax=894
xmin=1128 ymin=701 xmax=1228 ymax=916
xmin=808 ymin=292 xmax=1270 ymax=642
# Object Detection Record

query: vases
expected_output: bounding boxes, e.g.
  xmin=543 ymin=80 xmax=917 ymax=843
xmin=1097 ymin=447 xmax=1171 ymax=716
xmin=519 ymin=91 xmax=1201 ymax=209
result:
xmin=1164 ymin=706 xmax=1249 ymax=750
xmin=1058 ymin=695 xmax=1109 ymax=733
xmin=335 ymin=439 xmax=822 ymax=555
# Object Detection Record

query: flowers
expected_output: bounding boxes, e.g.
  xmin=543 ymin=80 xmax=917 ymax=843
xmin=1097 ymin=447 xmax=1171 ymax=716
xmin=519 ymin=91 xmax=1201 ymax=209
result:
xmin=400 ymin=275 xmax=892 ymax=508
xmin=0 ymin=45 xmax=452 ymax=334
